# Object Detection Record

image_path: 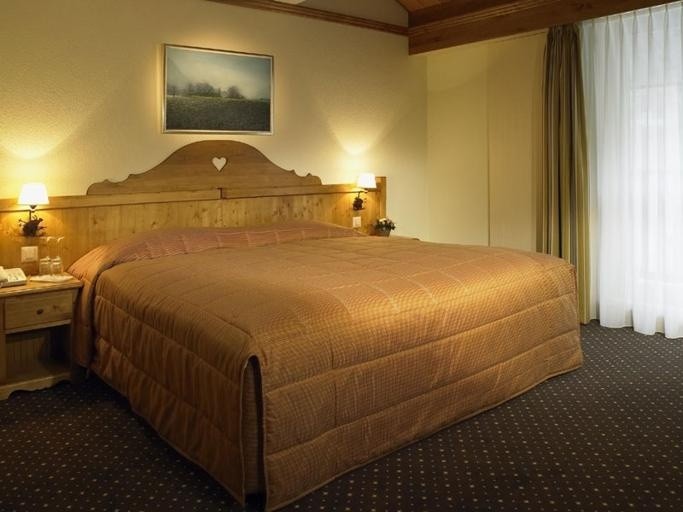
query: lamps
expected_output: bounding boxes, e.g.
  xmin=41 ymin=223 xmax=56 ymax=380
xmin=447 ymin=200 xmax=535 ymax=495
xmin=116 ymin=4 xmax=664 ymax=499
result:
xmin=350 ymin=172 xmax=377 ymax=211
xmin=15 ymin=182 xmax=50 ymax=238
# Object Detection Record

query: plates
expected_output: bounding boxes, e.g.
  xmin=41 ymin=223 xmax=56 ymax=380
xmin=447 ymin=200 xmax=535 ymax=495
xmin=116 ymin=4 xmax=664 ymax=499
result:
xmin=29 ymin=274 xmax=74 ymax=282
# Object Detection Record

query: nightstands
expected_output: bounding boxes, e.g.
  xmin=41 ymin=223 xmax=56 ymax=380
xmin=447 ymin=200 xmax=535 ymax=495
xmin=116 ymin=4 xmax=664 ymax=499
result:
xmin=0 ymin=272 xmax=84 ymax=400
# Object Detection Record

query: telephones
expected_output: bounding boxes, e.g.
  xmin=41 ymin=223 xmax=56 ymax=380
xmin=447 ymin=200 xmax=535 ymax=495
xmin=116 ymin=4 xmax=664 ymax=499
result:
xmin=0 ymin=265 xmax=28 ymax=288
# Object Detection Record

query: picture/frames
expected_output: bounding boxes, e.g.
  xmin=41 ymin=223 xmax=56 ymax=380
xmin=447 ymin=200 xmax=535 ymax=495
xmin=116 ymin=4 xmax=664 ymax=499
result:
xmin=159 ymin=43 xmax=274 ymax=137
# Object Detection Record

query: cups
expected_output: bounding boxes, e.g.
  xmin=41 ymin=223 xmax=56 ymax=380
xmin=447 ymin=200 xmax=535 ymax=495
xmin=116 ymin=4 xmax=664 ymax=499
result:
xmin=39 ymin=256 xmax=63 ymax=278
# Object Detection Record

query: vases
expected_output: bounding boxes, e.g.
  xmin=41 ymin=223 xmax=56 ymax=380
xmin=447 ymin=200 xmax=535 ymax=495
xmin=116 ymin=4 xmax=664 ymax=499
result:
xmin=375 ymin=230 xmax=390 ymax=237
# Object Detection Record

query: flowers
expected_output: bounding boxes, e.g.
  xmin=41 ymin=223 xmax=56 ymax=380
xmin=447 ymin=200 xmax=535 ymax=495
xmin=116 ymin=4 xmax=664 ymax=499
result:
xmin=373 ymin=218 xmax=395 ymax=231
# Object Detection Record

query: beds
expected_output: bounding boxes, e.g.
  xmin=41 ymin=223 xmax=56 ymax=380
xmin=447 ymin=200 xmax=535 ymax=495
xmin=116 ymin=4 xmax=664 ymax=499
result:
xmin=67 ymin=229 xmax=582 ymax=510
